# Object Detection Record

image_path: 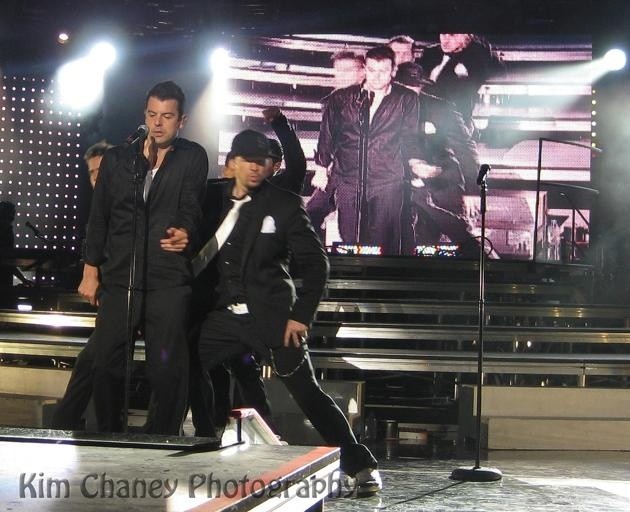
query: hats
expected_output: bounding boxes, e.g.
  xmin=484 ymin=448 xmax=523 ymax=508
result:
xmin=231 ymin=130 xmax=279 ymax=159
xmin=396 ymin=61 xmax=435 ymax=88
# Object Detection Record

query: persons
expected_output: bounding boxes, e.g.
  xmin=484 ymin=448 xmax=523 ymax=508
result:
xmin=53 ymin=80 xmax=383 ymax=494
xmin=297 ymin=17 xmax=506 ymax=257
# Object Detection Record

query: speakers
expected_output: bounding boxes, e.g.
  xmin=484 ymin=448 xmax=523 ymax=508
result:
xmin=266 ymin=380 xmax=364 ymax=450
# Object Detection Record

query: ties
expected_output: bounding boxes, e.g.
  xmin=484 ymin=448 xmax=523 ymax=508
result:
xmin=190 ymin=195 xmax=252 ymax=278
xmin=369 ymin=92 xmax=376 ymax=105
xmin=429 ymin=54 xmax=451 ymax=84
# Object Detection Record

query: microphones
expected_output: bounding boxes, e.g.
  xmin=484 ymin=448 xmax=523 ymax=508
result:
xmin=476 ymin=162 xmax=493 ymax=184
xmin=354 ymin=82 xmax=372 ymax=109
xmin=125 ymin=124 xmax=150 ymax=146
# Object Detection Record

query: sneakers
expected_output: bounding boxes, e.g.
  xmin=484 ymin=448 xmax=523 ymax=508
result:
xmin=355 ymin=468 xmax=382 ymax=492
